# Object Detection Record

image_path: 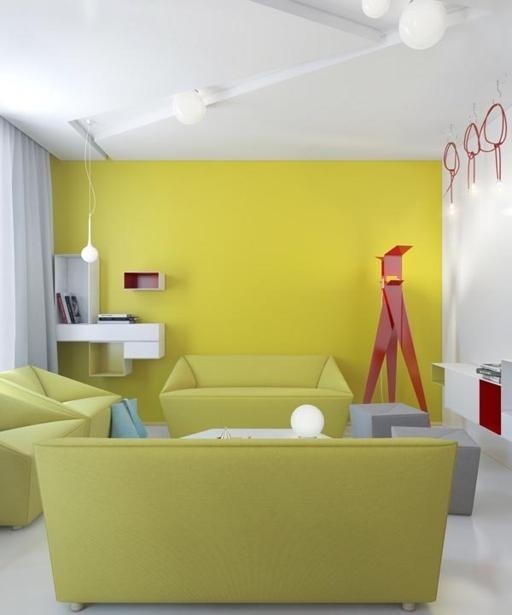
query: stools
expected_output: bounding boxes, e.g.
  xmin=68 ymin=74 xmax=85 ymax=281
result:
xmin=390 ymin=425 xmax=481 ymax=516
xmin=348 ymin=401 xmax=430 ymax=439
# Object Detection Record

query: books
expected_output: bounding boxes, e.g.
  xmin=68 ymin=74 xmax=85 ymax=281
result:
xmin=480 ymin=373 xmax=501 ymax=384
xmin=476 ymin=367 xmax=502 ymax=377
xmin=98 ymin=317 xmax=137 ymax=321
xmin=97 ymin=314 xmax=136 ymax=317
xmin=97 ymin=320 xmax=136 ymax=324
xmin=480 ymin=362 xmax=501 ymax=373
xmin=55 ymin=292 xmax=82 ymax=324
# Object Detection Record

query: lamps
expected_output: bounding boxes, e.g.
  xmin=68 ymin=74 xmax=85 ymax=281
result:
xmin=361 ymin=0 xmax=449 ymax=50
xmin=172 ymin=90 xmax=205 ymax=126
xmin=80 ymin=116 xmax=100 ymax=263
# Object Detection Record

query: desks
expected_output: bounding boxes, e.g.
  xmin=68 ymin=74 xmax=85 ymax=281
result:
xmin=180 ymin=426 xmax=330 ymax=441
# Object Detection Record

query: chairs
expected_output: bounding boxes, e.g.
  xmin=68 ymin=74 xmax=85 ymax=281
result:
xmin=0 ymin=366 xmax=123 ymax=530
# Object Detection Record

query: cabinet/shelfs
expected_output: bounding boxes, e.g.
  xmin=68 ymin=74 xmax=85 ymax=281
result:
xmin=430 ymin=358 xmax=512 ymax=468
xmin=53 ymin=253 xmax=166 ymax=378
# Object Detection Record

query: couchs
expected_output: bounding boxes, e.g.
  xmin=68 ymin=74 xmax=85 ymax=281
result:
xmin=157 ymin=353 xmax=356 ymax=432
xmin=36 ymin=435 xmax=459 ymax=611
